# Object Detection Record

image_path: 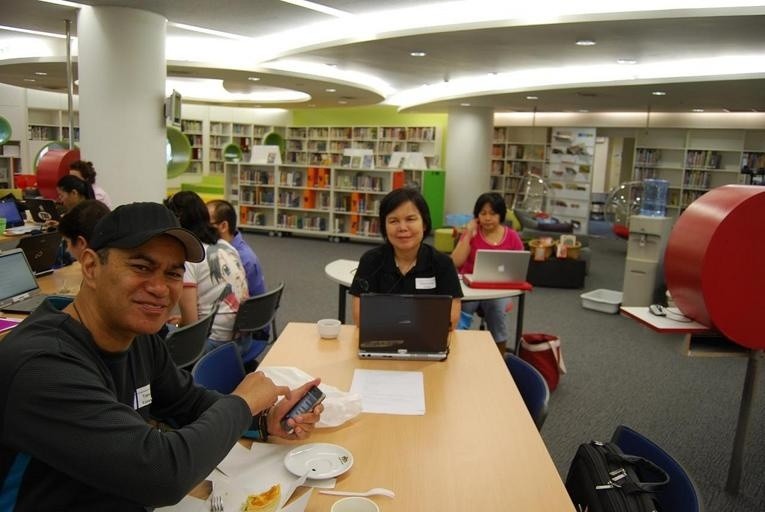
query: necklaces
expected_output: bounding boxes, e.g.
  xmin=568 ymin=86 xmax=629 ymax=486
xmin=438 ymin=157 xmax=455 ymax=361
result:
xmin=481 ymin=228 xmax=503 ymax=245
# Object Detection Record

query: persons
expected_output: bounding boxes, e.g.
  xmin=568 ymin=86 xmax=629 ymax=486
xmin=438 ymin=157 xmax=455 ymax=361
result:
xmin=164 ymin=194 xmax=269 ymax=373
xmin=46 ymin=159 xmax=110 ymax=269
xmin=346 ymin=184 xmax=463 ymax=333
xmin=451 ymin=191 xmax=521 ymax=360
xmin=1 ymin=200 xmax=325 ymax=512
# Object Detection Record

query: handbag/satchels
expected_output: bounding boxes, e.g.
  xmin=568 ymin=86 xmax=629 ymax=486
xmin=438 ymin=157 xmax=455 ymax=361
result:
xmin=519 ymin=330 xmax=564 ymax=391
xmin=565 ymin=440 xmax=674 ymax=511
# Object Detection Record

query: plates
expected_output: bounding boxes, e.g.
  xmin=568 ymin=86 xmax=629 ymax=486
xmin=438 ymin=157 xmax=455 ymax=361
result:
xmin=284 ymin=442 xmax=354 ymax=479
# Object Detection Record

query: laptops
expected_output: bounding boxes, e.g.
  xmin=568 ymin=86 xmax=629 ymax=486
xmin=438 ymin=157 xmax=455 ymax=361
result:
xmin=17 ymin=230 xmax=60 ymax=276
xmin=463 ymin=249 xmax=531 ymax=284
xmin=0 ymin=248 xmax=48 ymax=313
xmin=0 ymin=197 xmax=47 ymax=235
xmin=24 ymin=198 xmax=62 ymax=223
xmin=358 ymin=292 xmax=453 ymax=361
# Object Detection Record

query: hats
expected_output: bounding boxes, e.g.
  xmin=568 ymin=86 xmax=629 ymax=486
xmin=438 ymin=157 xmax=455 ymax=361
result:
xmin=92 ymin=200 xmax=205 ymax=262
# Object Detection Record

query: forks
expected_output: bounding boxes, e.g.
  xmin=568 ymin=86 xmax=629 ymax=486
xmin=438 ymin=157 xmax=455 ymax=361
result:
xmin=319 ymin=488 xmax=395 ymax=498
xmin=209 ymin=495 xmax=224 ymax=512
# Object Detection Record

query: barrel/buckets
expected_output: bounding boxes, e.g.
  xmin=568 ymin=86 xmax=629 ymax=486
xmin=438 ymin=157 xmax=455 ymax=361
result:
xmin=639 ymin=178 xmax=670 ymax=216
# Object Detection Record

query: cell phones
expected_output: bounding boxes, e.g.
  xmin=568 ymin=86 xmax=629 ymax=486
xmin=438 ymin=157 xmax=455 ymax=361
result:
xmin=279 ymin=385 xmax=326 ymax=434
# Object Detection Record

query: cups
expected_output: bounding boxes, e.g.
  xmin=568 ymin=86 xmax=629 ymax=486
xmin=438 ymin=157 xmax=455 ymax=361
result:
xmin=330 ymin=496 xmax=380 ymax=512
xmin=317 ymin=318 xmax=342 ymax=338
xmin=0 ymin=217 xmax=6 ymax=235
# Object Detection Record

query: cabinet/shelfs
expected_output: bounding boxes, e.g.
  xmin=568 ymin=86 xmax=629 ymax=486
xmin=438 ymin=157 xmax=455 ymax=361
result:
xmin=28 ymin=106 xmax=78 ymax=143
xmin=491 ymin=128 xmax=594 ymax=235
xmin=0 ymin=142 xmax=21 ymax=186
xmin=176 ymin=119 xmax=436 ymax=244
xmin=627 ymin=132 xmax=765 ymax=214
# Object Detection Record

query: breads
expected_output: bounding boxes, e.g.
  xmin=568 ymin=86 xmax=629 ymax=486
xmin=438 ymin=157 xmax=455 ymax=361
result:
xmin=244 ymin=482 xmax=280 ymax=512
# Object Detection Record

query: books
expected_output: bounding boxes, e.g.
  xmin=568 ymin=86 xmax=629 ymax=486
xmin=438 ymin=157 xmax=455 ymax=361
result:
xmin=181 ymin=120 xmax=204 ymax=173
xmin=226 ymin=164 xmax=274 ymax=226
xmin=490 ymin=126 xmax=541 ymax=213
xmin=630 ymin=148 xmax=678 ymax=214
xmin=276 ymin=167 xmax=331 ymax=233
xmin=288 ymin=128 xmax=352 ymax=167
xmin=335 ymin=171 xmax=422 ymax=238
xmin=680 ymin=150 xmax=721 ymax=214
xmin=341 ymin=127 xmax=436 ymax=170
xmin=209 ymin=123 xmax=269 ymax=175
xmin=28 ymin=126 xmax=79 ymax=142
xmin=548 ymin=135 xmax=590 ymax=209
xmin=738 ymin=152 xmax=765 ymax=187
xmin=1 ymin=144 xmax=21 ymax=188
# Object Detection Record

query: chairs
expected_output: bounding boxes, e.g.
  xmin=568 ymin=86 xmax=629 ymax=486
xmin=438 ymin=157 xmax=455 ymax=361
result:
xmin=502 ymin=355 xmax=552 ymax=433
xmin=188 ymin=341 xmax=258 ymax=397
xmin=239 ymin=281 xmax=296 ymax=361
xmin=167 ymin=303 xmax=221 ymax=369
xmin=16 ymin=231 xmax=64 ymax=271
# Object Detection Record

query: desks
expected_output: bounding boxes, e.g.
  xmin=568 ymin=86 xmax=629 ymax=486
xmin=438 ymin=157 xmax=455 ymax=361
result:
xmin=323 ymin=254 xmax=528 ymax=350
xmin=527 ymin=246 xmax=591 ymax=289
xmin=0 ymin=261 xmax=80 ymax=346
xmin=0 ymin=213 xmax=69 ymax=267
xmin=152 ymin=321 xmax=577 ymax=512
